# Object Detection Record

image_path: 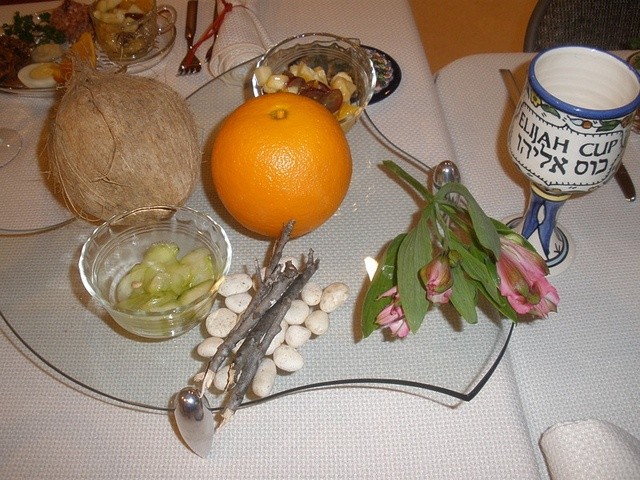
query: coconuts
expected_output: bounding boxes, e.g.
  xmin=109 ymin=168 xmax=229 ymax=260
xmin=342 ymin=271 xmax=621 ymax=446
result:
xmin=46 ymin=53 xmax=201 ymax=226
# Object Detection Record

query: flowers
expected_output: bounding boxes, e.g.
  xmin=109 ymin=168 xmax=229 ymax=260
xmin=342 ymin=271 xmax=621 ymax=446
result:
xmin=359 ymin=159 xmax=561 ymax=341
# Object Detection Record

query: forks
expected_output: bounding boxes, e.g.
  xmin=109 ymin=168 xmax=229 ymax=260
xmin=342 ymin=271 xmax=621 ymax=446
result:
xmin=176 ymin=0 xmax=201 ymax=77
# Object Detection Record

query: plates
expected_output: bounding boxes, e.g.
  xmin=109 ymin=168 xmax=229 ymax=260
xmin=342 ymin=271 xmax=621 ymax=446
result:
xmin=0 ymin=8 xmax=177 ymax=98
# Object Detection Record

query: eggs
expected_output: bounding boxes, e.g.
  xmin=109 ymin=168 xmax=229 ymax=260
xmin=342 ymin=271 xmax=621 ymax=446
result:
xmin=18 ymin=61 xmax=57 ymax=89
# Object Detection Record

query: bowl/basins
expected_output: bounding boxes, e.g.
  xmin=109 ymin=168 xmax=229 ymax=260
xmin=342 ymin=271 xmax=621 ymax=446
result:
xmin=251 ymin=32 xmax=376 ymax=134
xmin=79 ymin=205 xmax=232 ymax=340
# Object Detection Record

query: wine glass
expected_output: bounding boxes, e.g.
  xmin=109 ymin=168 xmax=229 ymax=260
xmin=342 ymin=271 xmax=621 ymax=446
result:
xmin=501 ymin=45 xmax=640 ymax=278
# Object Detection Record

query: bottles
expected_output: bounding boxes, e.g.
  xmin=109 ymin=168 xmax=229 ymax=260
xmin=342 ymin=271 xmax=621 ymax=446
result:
xmin=431 ymin=159 xmax=461 ymax=239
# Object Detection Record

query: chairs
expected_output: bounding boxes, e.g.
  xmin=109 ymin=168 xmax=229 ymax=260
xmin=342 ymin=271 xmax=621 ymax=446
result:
xmin=524 ymin=0 xmax=640 ymax=52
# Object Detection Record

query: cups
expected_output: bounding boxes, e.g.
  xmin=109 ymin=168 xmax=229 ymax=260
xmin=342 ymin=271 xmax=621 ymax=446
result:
xmin=627 ymin=51 xmax=640 ymax=135
xmin=89 ymin=0 xmax=177 ymax=62
